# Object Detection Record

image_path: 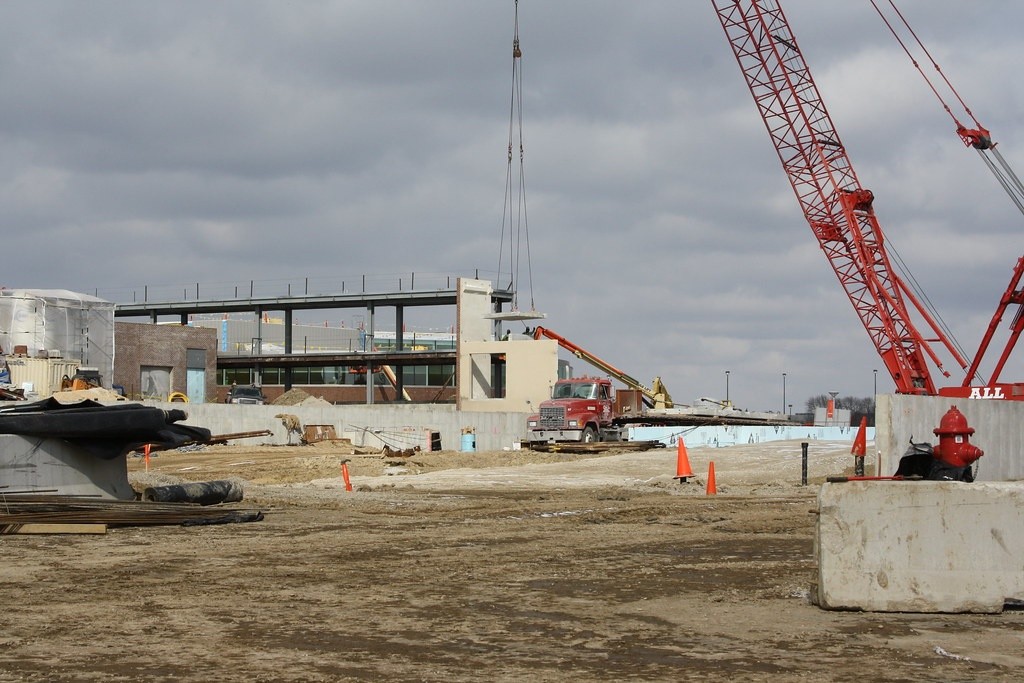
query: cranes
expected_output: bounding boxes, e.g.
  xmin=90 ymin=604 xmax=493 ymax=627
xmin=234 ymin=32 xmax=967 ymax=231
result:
xmin=712 ymin=0 xmax=1024 ymax=401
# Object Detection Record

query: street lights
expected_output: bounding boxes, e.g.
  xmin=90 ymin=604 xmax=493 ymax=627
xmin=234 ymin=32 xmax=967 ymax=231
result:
xmin=873 ymin=369 xmax=877 ymax=396
xmin=782 ymin=373 xmax=787 ymax=415
xmin=788 ymin=404 xmax=793 ymax=419
xmin=726 ymin=370 xmax=730 ymax=405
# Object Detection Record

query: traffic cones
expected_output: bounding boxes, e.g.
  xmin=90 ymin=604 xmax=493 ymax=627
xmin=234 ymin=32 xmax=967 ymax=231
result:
xmin=850 ymin=415 xmax=867 ymax=457
xmin=671 ymin=436 xmax=695 ymax=479
xmin=706 ymin=461 xmax=717 ymax=495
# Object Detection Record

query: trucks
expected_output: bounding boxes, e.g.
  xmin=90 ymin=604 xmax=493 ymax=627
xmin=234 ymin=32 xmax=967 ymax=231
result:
xmin=527 ymin=374 xmax=642 ymax=447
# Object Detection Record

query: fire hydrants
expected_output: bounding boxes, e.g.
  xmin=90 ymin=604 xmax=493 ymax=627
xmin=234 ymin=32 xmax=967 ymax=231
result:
xmin=924 ymin=403 xmax=984 ymax=482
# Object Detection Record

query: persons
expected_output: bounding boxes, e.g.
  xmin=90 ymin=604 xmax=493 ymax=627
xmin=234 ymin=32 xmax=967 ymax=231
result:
xmin=597 ymin=387 xmax=604 ymax=398
xmin=227 ymin=383 xmax=236 ymax=398
xmin=250 ymin=383 xmax=262 ymax=396
xmin=502 ymin=329 xmax=510 ymax=340
xmin=61 ymin=375 xmax=73 ymax=391
xmin=522 ymin=326 xmax=535 ymax=336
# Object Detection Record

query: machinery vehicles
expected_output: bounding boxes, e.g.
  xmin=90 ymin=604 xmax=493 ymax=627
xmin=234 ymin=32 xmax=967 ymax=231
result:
xmin=528 ymin=324 xmax=677 ymax=413
xmin=59 ymin=368 xmax=128 ymax=403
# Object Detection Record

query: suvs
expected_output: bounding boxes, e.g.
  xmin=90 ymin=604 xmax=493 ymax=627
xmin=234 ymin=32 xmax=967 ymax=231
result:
xmin=223 ymin=382 xmax=268 ymax=407
